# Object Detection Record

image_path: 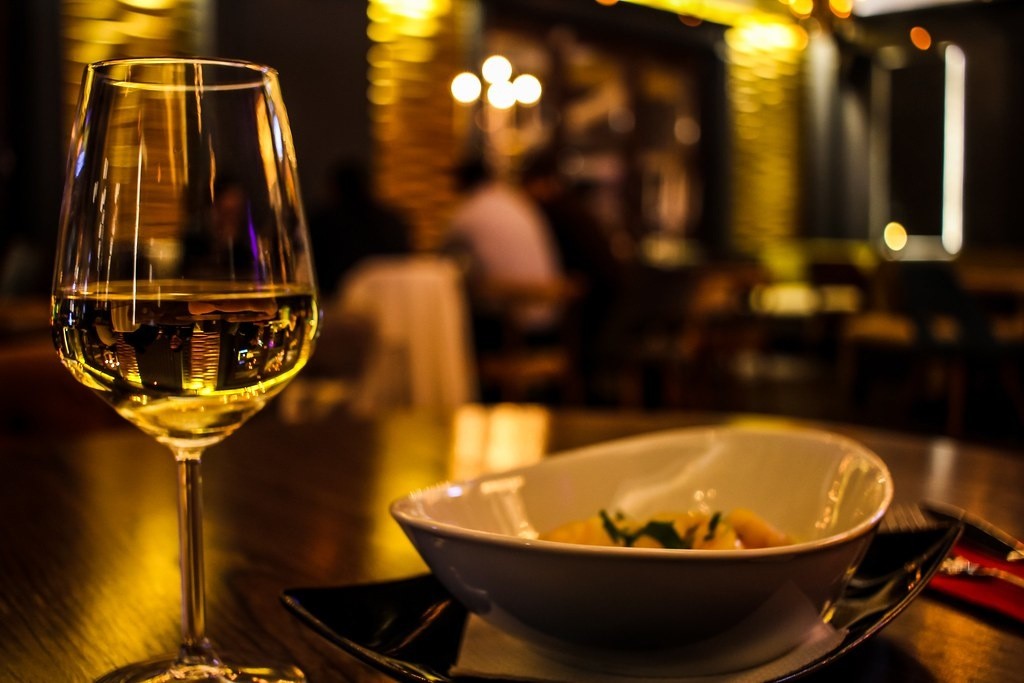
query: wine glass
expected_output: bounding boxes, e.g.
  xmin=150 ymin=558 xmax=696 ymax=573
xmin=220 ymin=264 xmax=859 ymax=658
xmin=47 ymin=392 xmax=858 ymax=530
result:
xmin=50 ymin=57 xmax=320 ymax=683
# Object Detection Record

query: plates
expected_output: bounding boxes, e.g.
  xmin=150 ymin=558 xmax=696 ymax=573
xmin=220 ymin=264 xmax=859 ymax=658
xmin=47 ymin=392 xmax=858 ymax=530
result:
xmin=277 ymin=516 xmax=963 ymax=683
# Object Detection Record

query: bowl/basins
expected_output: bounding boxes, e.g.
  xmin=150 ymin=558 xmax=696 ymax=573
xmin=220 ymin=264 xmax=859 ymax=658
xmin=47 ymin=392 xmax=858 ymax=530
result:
xmin=389 ymin=418 xmax=893 ymax=674
